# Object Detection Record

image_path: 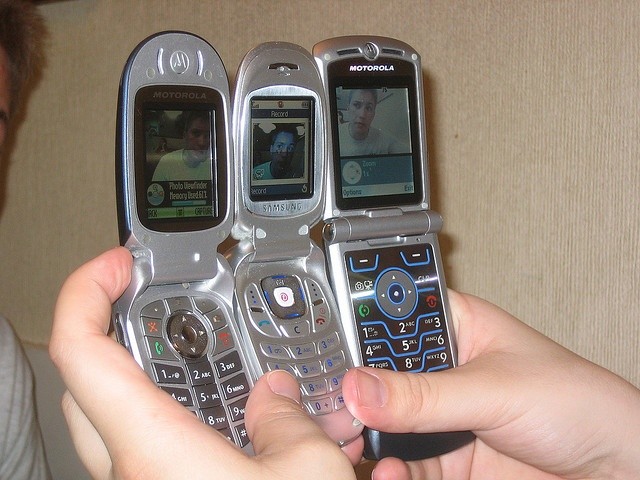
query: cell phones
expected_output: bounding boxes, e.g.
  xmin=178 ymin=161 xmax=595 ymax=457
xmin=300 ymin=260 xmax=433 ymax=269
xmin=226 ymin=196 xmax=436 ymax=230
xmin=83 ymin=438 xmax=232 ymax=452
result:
xmin=111 ymin=30 xmax=257 ymax=458
xmin=222 ymin=41 xmax=364 ymax=447
xmin=311 ymin=34 xmax=476 ymax=462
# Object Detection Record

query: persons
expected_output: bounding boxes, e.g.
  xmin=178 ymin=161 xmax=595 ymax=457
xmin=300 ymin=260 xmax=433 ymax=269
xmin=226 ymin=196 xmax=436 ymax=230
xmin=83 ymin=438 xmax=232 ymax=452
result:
xmin=252 ymin=130 xmax=300 ymax=180
xmin=0 ymin=0 xmax=53 ymax=479
xmin=48 ymin=245 xmax=639 ymax=480
xmin=338 ymin=89 xmax=410 ymax=156
xmin=152 ymin=113 xmax=212 ymax=182
